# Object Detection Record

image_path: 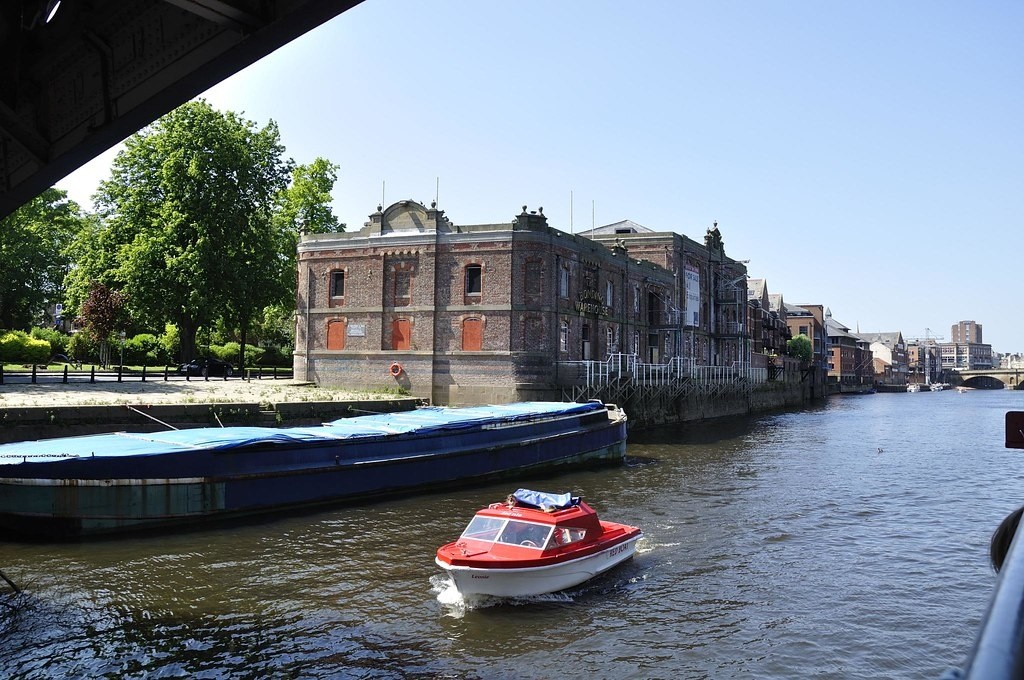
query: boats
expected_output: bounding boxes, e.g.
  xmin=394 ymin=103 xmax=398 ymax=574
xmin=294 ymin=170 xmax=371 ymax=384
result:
xmin=930 ymin=383 xmax=943 ymax=392
xmin=957 ymin=388 xmax=967 ymax=393
xmin=907 ymin=382 xmax=921 ymax=393
xmin=1 ymin=399 xmax=627 ymax=532
xmin=435 ymin=489 xmax=644 ymax=602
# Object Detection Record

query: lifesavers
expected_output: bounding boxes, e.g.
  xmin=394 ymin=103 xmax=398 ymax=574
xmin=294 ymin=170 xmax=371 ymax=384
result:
xmin=390 ymin=362 xmax=403 ymax=377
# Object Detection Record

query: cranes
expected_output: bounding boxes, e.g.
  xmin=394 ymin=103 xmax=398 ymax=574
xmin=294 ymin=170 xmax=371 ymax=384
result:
xmin=903 ymin=328 xmax=945 ymax=341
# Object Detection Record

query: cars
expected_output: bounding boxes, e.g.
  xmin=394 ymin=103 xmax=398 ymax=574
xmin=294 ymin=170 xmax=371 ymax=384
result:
xmin=177 ymin=356 xmax=234 ymax=377
